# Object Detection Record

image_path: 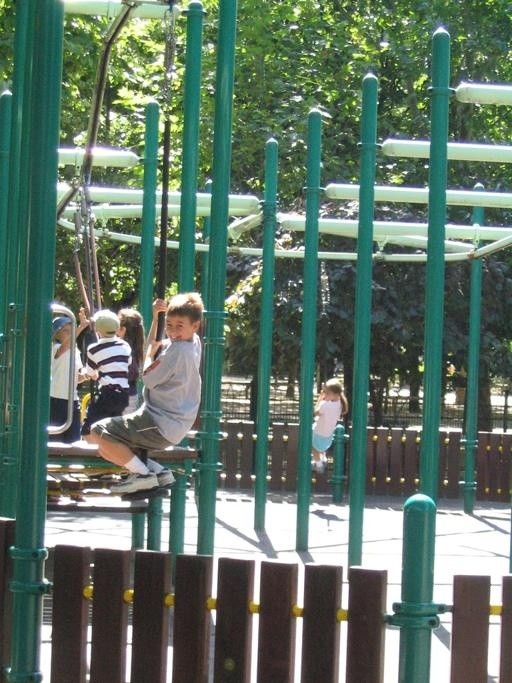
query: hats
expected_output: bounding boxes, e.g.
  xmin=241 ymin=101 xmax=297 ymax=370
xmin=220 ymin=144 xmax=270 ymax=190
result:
xmin=92 ymin=309 xmax=120 ymax=337
xmin=52 ymin=317 xmax=74 ymax=338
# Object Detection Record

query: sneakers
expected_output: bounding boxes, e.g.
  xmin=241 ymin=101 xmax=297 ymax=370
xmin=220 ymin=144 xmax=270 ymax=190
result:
xmin=110 ymin=466 xmax=176 ymax=495
xmin=311 ymin=453 xmax=328 ymax=474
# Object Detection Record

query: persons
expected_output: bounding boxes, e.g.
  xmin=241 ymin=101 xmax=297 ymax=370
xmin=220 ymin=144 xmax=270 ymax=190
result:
xmin=90 ymin=292 xmax=204 ymax=494
xmin=311 ymin=378 xmax=348 ymax=473
xmin=48 ymin=306 xmax=92 ymax=473
xmin=80 ymin=310 xmax=133 ymax=445
xmin=118 ymin=308 xmax=145 ymax=415
